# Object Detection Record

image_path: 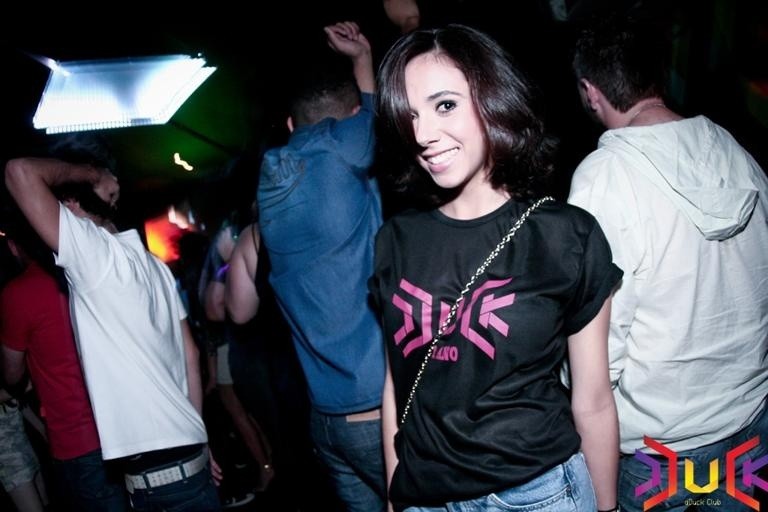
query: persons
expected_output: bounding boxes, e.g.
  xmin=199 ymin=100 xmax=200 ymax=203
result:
xmin=1 ymin=0 xmax=767 ymax=512
